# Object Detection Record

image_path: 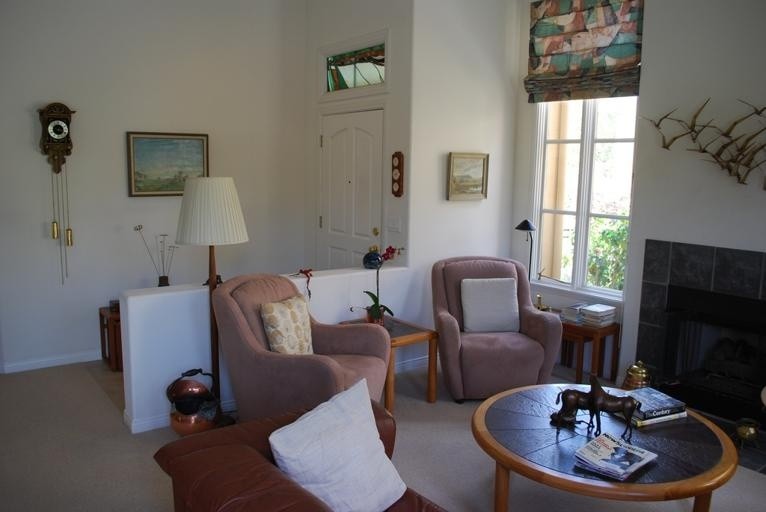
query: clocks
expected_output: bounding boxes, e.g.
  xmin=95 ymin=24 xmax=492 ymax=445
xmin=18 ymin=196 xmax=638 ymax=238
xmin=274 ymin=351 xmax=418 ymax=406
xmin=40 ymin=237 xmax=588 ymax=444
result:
xmin=35 ymin=103 xmax=76 ymax=287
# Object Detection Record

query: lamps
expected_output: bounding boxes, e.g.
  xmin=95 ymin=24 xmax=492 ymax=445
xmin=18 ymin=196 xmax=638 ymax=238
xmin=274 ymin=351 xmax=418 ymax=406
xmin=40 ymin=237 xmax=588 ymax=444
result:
xmin=173 ymin=177 xmax=247 ymax=429
xmin=514 ymin=218 xmax=536 ymax=283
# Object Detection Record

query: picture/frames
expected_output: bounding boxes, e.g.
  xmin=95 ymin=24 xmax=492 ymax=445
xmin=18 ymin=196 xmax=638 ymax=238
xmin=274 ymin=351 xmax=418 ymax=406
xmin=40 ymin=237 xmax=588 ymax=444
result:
xmin=445 ymin=150 xmax=489 ymax=204
xmin=125 ymin=130 xmax=209 ymax=198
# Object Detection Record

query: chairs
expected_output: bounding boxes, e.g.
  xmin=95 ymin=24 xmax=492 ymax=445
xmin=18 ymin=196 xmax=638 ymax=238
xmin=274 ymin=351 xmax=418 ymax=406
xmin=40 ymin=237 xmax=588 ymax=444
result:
xmin=210 ymin=274 xmax=393 ymax=428
xmin=427 ymin=254 xmax=562 ymax=401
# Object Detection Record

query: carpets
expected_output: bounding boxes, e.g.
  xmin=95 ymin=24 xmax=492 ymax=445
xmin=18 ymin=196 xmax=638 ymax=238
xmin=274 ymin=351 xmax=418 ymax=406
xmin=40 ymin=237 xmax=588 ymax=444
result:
xmin=390 ymin=365 xmax=765 ymax=512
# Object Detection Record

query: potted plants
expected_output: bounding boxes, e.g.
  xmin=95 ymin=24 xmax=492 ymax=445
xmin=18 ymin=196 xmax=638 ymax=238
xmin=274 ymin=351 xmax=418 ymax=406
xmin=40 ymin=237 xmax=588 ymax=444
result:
xmin=363 ymin=306 xmax=385 ymax=327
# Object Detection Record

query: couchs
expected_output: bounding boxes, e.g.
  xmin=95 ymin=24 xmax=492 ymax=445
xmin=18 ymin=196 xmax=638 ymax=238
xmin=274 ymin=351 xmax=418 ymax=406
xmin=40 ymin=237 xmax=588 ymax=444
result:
xmin=151 ymin=406 xmax=450 ymax=511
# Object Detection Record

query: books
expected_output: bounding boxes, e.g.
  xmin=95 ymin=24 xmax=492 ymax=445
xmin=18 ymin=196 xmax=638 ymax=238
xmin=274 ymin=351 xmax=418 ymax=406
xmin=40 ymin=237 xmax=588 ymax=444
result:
xmin=569 ymin=433 xmax=658 ymax=483
xmin=614 ymin=411 xmax=688 ymax=427
xmin=615 ymin=387 xmax=689 ymax=420
xmin=558 ymin=300 xmax=616 ymax=329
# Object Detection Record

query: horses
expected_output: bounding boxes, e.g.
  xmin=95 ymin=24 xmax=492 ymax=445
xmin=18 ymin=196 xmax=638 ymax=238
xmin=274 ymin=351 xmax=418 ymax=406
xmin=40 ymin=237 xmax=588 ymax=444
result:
xmin=556 ymin=389 xmax=595 ymax=433
xmin=588 ymin=373 xmax=642 ymax=441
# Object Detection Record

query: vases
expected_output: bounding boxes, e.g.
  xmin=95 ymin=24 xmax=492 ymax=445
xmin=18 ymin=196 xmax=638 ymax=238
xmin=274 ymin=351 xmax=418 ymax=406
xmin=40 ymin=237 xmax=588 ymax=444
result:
xmin=156 ymin=274 xmax=171 ymax=287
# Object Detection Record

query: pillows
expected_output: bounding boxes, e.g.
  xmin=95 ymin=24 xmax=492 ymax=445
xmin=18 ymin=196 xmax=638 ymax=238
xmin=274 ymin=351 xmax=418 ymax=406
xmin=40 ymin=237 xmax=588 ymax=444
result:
xmin=267 ymin=378 xmax=409 ymax=512
xmin=458 ymin=276 xmax=519 ymax=334
xmin=257 ymin=295 xmax=314 ymax=357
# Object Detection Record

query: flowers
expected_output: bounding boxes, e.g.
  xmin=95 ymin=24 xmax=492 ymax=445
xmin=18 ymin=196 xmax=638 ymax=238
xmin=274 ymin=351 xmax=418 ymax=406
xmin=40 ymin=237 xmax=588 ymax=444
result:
xmin=348 ymin=241 xmax=406 ymax=318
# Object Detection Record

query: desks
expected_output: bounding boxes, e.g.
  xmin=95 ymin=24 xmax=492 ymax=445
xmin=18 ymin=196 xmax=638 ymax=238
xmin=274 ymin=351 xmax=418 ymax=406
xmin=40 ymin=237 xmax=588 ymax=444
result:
xmin=338 ymin=314 xmax=439 ymax=414
xmin=98 ymin=305 xmax=123 ymax=374
xmin=534 ymin=303 xmax=622 ymax=386
